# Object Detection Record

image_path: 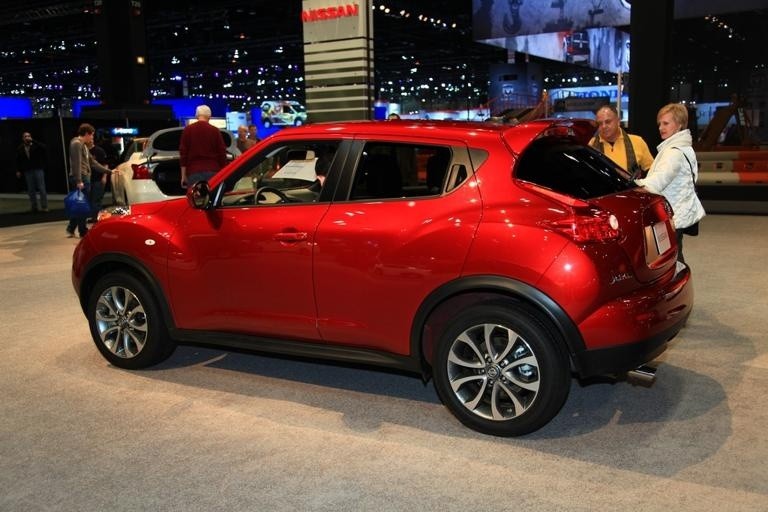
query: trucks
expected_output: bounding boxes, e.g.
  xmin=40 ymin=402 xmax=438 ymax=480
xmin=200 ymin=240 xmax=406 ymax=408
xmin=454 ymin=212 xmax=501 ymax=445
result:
xmin=260 ymin=100 xmax=307 ymax=128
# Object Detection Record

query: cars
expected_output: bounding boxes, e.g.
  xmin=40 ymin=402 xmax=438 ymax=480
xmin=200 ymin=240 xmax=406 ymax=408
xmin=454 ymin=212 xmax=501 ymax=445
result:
xmin=71 ymin=116 xmax=690 ymax=437
xmin=107 ymin=123 xmax=245 ymax=212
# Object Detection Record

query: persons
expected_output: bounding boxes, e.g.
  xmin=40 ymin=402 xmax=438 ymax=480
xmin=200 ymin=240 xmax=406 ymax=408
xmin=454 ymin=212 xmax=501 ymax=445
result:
xmin=388 ymin=113 xmax=400 ymax=120
xmin=502 ymin=116 xmax=519 ymax=126
xmin=85 ymin=134 xmax=110 ymax=223
xmin=247 ymin=124 xmax=261 ymax=143
xmin=14 ymin=131 xmax=50 ymax=213
xmin=236 ymin=125 xmax=256 ymax=153
xmin=64 ymin=123 xmax=120 ymax=239
xmin=178 ymin=103 xmax=228 ymax=191
xmin=633 ymin=103 xmax=706 ymax=268
xmin=314 ymin=152 xmax=336 ymax=188
xmin=587 ymin=103 xmax=655 ymax=181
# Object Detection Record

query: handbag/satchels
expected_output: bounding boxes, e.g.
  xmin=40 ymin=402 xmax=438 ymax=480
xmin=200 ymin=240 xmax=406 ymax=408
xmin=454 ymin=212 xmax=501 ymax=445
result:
xmin=64 ymin=188 xmax=91 ymax=217
xmin=684 ymin=221 xmax=699 ymax=237
